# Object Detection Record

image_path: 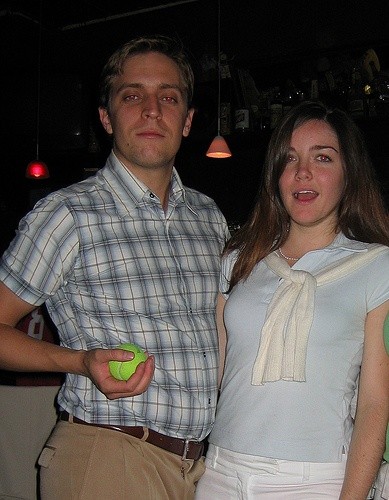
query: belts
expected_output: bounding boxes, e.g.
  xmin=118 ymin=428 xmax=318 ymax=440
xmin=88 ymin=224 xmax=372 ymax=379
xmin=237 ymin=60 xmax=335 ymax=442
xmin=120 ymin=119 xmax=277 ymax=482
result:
xmin=60 ymin=409 xmax=205 ymax=461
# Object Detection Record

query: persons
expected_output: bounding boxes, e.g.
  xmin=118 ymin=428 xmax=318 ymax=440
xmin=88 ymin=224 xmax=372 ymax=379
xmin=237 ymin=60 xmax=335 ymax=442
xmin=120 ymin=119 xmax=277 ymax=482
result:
xmin=195 ymin=99 xmax=389 ymax=500
xmin=0 ymin=37 xmax=233 ymax=500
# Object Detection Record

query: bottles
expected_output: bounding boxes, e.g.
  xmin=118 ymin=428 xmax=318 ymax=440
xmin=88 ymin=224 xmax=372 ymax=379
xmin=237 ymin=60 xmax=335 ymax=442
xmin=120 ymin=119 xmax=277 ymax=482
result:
xmin=216 ymin=39 xmax=388 ymax=136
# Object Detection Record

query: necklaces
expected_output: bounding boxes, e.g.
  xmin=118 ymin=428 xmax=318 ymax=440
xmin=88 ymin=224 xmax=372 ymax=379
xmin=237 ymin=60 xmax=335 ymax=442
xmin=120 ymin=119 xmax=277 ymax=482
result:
xmin=277 ymin=247 xmax=298 ymax=261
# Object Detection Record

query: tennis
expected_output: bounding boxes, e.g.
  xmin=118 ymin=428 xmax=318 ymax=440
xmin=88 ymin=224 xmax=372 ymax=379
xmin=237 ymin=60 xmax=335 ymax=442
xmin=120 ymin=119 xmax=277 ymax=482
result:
xmin=108 ymin=343 xmax=148 ymax=382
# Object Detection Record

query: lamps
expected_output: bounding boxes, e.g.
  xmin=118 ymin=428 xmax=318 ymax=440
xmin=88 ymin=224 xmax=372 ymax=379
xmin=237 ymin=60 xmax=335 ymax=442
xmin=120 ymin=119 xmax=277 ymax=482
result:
xmin=206 ymin=0 xmax=233 ymax=159
xmin=24 ymin=23 xmax=50 ymax=181
